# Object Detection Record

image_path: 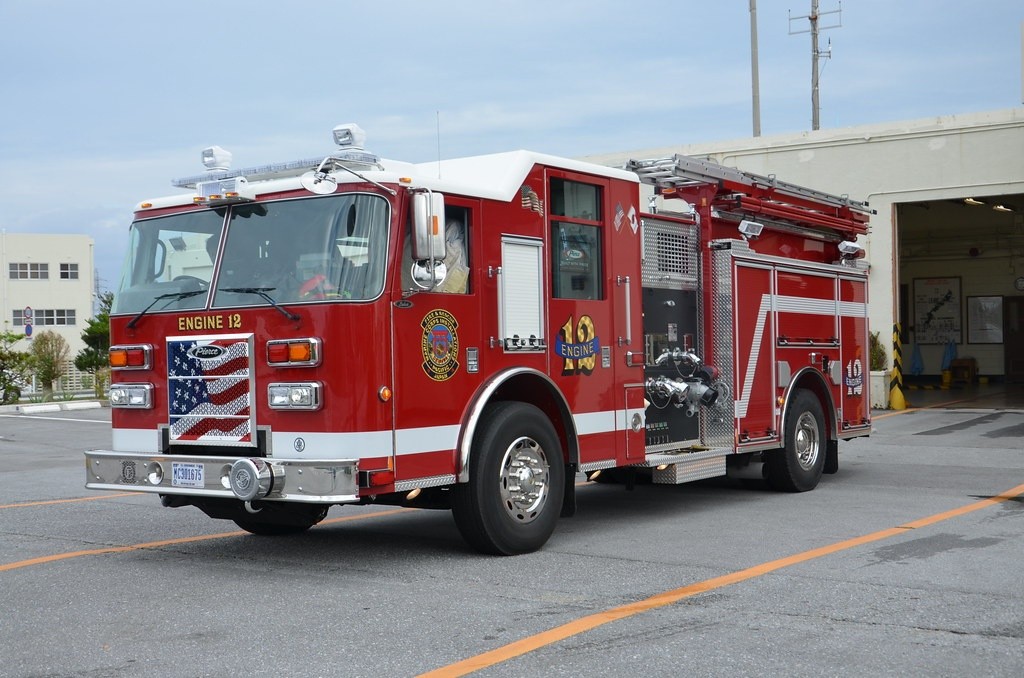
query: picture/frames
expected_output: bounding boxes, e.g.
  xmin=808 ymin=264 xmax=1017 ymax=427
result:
xmin=966 ymin=295 xmax=1004 ymax=345
xmin=912 ymin=276 xmax=963 ymax=345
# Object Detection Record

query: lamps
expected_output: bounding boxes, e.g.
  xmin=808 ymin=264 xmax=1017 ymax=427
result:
xmin=963 ymin=198 xmax=986 ymax=207
xmin=993 ymin=203 xmax=1017 ymax=213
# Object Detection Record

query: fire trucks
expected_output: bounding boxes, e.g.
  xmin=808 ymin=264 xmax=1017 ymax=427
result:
xmin=85 ymin=124 xmax=878 ymax=558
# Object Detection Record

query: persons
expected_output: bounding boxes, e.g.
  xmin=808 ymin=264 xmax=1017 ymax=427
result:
xmin=205 ymin=232 xmax=247 ymax=289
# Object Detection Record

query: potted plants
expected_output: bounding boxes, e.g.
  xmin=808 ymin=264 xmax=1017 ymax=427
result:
xmin=869 ymin=331 xmax=892 ymax=409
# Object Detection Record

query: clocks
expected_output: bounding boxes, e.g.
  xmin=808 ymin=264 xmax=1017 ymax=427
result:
xmin=1014 ymin=277 xmax=1024 ymax=291
